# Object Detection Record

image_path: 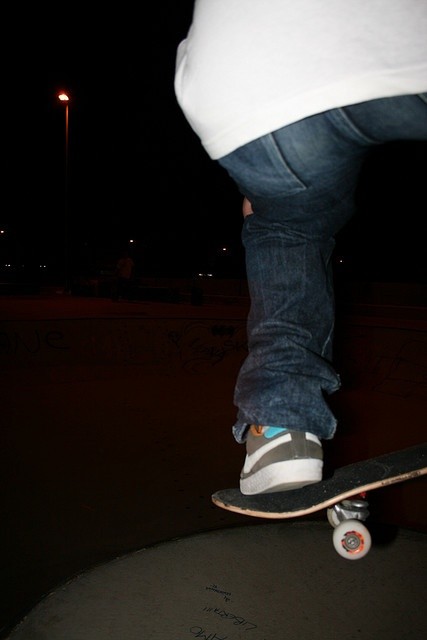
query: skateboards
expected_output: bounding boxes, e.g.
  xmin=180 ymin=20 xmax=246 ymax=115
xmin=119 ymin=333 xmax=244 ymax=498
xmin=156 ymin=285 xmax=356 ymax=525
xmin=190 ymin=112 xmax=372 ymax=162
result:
xmin=213 ymin=440 xmax=427 ymax=560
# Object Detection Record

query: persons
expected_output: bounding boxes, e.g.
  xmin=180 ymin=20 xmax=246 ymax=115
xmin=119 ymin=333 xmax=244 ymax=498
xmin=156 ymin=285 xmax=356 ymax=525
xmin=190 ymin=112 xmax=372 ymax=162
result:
xmin=172 ymin=0 xmax=427 ymax=495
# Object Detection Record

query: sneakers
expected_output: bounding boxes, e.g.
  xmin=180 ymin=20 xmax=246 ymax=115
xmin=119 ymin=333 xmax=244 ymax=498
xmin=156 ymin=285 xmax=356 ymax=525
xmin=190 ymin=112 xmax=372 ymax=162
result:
xmin=239 ymin=423 xmax=324 ymax=496
